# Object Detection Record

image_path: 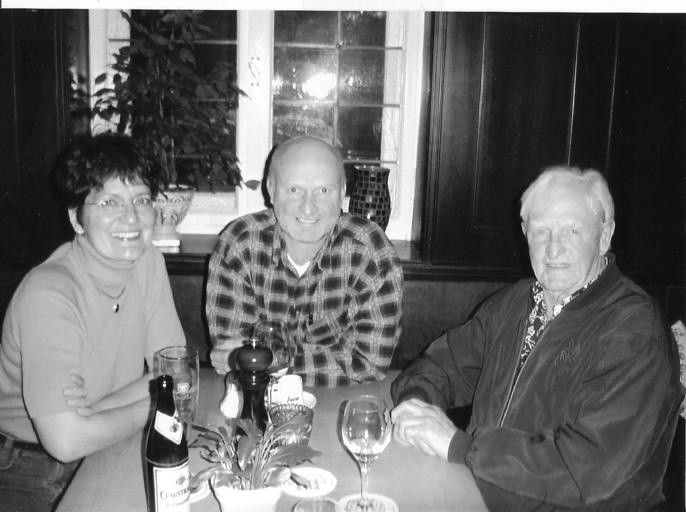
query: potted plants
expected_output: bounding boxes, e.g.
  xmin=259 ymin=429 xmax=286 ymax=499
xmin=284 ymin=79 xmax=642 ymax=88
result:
xmin=65 ymin=8 xmax=259 ymax=252
xmin=179 ymin=412 xmax=321 ymax=512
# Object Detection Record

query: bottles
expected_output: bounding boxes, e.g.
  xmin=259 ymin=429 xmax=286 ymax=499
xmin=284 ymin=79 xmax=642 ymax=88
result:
xmin=143 ymin=374 xmax=192 ymax=512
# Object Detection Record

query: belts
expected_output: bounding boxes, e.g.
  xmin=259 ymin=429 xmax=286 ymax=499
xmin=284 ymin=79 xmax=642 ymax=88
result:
xmin=0 ymin=435 xmax=46 ymax=452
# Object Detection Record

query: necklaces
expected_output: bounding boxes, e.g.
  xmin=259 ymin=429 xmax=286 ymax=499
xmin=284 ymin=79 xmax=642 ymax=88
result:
xmin=89 ymin=275 xmax=128 ymax=313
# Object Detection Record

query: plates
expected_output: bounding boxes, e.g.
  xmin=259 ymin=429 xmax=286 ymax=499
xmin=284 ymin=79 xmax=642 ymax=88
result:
xmin=265 ymin=391 xmax=318 ymax=410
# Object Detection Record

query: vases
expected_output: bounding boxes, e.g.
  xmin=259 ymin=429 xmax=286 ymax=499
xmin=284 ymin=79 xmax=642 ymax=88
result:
xmin=348 ymin=165 xmax=393 ymax=238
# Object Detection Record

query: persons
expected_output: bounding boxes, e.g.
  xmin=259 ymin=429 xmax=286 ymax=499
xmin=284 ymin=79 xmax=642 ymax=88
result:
xmin=203 ymin=132 xmax=408 ymax=387
xmin=0 ymin=129 xmax=197 ymax=512
xmin=387 ymin=164 xmax=686 ymax=512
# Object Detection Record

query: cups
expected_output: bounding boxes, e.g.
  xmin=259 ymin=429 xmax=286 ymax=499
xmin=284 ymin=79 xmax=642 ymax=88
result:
xmin=269 ymin=375 xmax=314 ymax=457
xmin=159 ymin=346 xmax=200 ymax=427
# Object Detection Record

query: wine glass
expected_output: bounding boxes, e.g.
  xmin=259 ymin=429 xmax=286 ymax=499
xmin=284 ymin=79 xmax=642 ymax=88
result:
xmin=340 ymin=395 xmax=392 ymax=511
xmin=252 ymin=318 xmax=292 ymax=410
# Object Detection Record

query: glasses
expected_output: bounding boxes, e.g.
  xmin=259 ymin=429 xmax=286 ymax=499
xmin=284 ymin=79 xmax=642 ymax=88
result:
xmin=84 ymin=196 xmax=158 ymax=210
